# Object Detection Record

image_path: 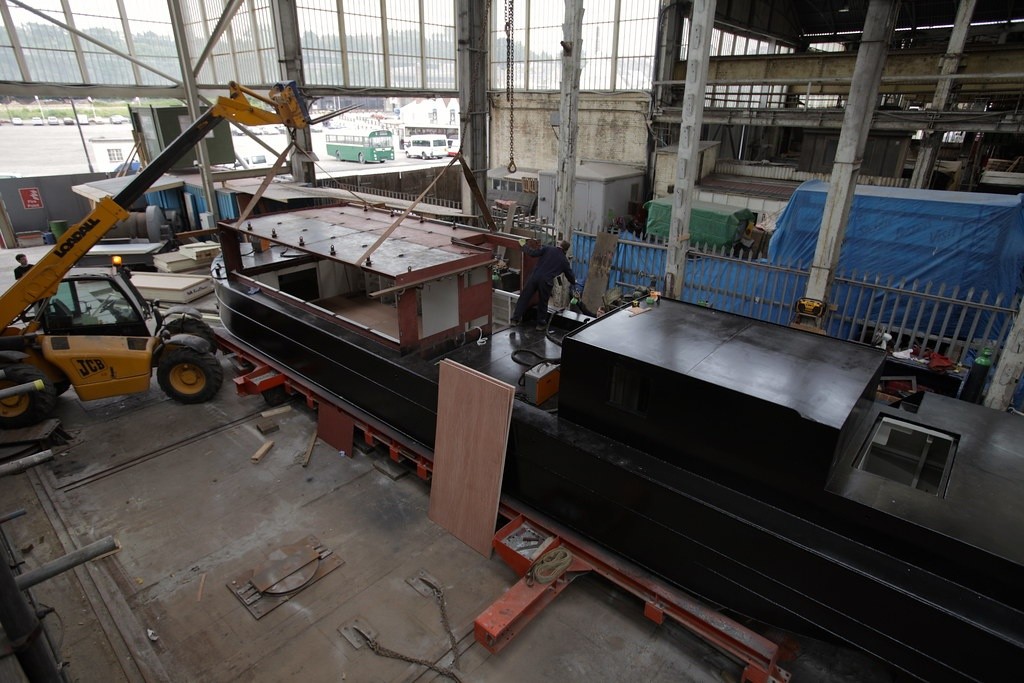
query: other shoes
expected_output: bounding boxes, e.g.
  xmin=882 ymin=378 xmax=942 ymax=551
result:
xmin=536 ymin=324 xmax=550 ymax=331
xmin=511 ymin=319 xmax=520 ymax=327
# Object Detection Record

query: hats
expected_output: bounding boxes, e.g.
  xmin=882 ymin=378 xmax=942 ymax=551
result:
xmin=561 ymin=241 xmax=570 ymax=250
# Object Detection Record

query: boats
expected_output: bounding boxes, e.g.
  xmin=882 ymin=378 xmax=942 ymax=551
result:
xmin=211 ymin=189 xmax=1024 ymax=682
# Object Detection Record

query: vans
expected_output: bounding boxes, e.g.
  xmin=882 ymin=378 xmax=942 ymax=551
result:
xmin=404 ymin=135 xmax=449 ymax=158
xmin=77 ymin=113 xmax=90 ymax=125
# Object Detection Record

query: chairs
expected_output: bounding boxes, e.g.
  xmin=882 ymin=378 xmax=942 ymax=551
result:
xmin=52 ymin=298 xmax=99 ymax=325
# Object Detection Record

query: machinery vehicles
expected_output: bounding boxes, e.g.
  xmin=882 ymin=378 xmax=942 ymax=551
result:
xmin=0 ymin=80 xmax=363 ymax=433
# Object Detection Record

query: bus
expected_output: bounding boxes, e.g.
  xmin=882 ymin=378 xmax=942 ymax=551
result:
xmin=326 ymin=128 xmax=395 ymax=163
xmin=400 ymin=124 xmax=460 ymax=154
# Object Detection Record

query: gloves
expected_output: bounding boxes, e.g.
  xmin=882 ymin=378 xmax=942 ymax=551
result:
xmin=519 ymin=239 xmax=526 ymax=247
xmin=573 ymin=283 xmax=579 ymax=294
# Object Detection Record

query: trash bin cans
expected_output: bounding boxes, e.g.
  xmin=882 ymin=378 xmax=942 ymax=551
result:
xmin=44 ymin=232 xmax=55 ymax=245
xmin=49 ymin=219 xmax=68 ymax=240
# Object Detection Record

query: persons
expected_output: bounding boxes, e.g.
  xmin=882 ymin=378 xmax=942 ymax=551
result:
xmin=512 ymin=238 xmax=579 ymax=332
xmin=568 ymin=290 xmax=607 ymax=318
xmin=14 ymin=253 xmax=46 ymax=314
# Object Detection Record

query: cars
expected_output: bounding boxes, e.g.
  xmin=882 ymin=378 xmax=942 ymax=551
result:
xmin=11 ymin=117 xmax=24 ymax=125
xmin=48 ymin=116 xmax=61 ymax=125
xmin=110 ymin=115 xmax=123 ymax=124
xmin=95 ymin=116 xmax=105 ymax=123
xmin=31 ymin=117 xmax=44 ymax=124
xmin=233 ymin=120 xmax=324 ymax=135
xmin=63 ymin=116 xmax=75 ymax=125
